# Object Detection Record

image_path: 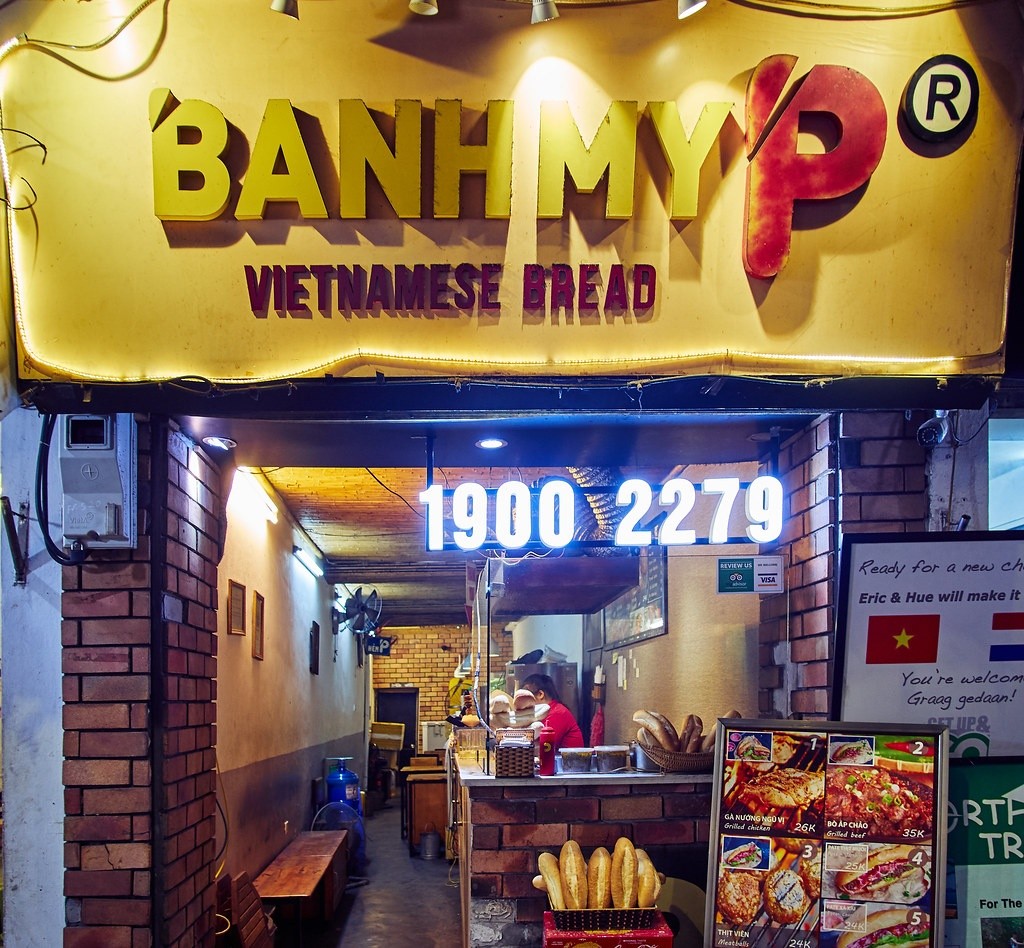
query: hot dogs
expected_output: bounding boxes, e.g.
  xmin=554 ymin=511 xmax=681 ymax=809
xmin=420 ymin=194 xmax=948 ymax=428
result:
xmin=837 ymin=909 xmax=931 ymax=948
xmin=833 ymin=743 xmax=865 ymax=762
xmin=727 ymin=842 xmax=761 ymax=866
xmin=738 ymin=740 xmax=771 ymax=760
xmin=834 ymin=844 xmax=923 ymax=895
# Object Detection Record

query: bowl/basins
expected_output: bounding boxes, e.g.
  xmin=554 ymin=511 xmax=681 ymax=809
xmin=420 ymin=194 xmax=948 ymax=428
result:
xmin=594 ymin=745 xmax=629 ymax=773
xmin=558 ymin=748 xmax=595 ymax=772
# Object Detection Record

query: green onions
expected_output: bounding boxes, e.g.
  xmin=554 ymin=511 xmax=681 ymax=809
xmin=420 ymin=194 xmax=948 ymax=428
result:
xmin=837 ymin=769 xmax=919 ymax=811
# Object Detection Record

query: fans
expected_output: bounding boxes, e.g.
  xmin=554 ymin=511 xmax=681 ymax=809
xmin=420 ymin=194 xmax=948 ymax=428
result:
xmin=333 ymin=583 xmax=382 ymax=636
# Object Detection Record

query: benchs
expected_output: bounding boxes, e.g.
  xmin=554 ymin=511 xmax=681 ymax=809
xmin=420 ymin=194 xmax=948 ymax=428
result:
xmin=254 ymin=829 xmax=349 ymax=923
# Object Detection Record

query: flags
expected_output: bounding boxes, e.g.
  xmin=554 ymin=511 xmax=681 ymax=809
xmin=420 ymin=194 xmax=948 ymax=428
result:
xmin=865 ymin=615 xmax=940 ymax=664
xmin=989 ymin=612 xmax=1024 ymax=661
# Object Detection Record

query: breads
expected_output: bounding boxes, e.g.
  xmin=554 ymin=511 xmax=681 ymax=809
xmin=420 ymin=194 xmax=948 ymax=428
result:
xmin=489 ymin=688 xmax=551 ymax=740
xmin=632 ymin=708 xmax=742 ymax=754
xmin=532 ymin=837 xmax=662 ymax=909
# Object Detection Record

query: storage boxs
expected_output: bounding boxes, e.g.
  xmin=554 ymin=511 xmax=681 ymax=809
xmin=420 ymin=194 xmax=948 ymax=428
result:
xmin=369 ymin=721 xmax=405 ymax=770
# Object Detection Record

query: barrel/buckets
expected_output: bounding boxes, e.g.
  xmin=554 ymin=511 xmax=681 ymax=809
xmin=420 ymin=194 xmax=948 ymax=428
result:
xmin=420 ymin=822 xmax=441 ymax=859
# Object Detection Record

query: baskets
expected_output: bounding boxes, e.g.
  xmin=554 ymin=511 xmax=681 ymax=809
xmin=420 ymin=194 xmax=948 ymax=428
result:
xmin=495 ymin=742 xmax=534 ymax=778
xmin=636 ymin=740 xmax=714 ymax=772
xmin=548 ymin=893 xmax=657 ymax=929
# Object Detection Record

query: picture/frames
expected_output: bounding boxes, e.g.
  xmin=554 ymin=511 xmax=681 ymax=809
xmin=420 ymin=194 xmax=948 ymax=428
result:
xmin=310 ymin=621 xmax=323 ymax=675
xmin=249 ymin=591 xmax=266 ymax=662
xmin=227 ymin=580 xmax=248 ymax=637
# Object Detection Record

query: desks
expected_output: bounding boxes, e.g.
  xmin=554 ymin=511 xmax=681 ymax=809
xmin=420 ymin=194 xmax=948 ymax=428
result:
xmin=400 ymin=766 xmax=454 ymax=839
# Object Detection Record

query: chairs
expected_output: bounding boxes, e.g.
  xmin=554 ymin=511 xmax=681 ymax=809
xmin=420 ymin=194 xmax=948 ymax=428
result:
xmin=409 ymin=749 xmax=447 ymax=767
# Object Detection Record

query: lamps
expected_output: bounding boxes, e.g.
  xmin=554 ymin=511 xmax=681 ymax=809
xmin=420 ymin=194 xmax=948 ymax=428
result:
xmin=451 ymin=632 xmax=502 ymax=677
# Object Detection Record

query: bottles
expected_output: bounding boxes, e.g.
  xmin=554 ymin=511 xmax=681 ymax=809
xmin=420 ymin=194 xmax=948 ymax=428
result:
xmin=540 ymin=720 xmax=556 ymax=776
xmin=327 ymin=760 xmax=361 ymax=820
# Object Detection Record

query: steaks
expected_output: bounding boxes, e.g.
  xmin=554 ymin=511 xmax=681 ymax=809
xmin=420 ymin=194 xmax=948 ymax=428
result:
xmin=763 ymin=869 xmax=808 ymax=924
xmin=799 ymin=848 xmax=822 ymax=899
xmin=717 ymin=871 xmax=760 ymax=926
xmin=742 ymin=767 xmax=823 ymax=808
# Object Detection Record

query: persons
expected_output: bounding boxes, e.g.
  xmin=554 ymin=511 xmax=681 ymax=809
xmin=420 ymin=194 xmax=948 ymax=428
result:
xmin=520 ymin=674 xmax=584 ymax=776
